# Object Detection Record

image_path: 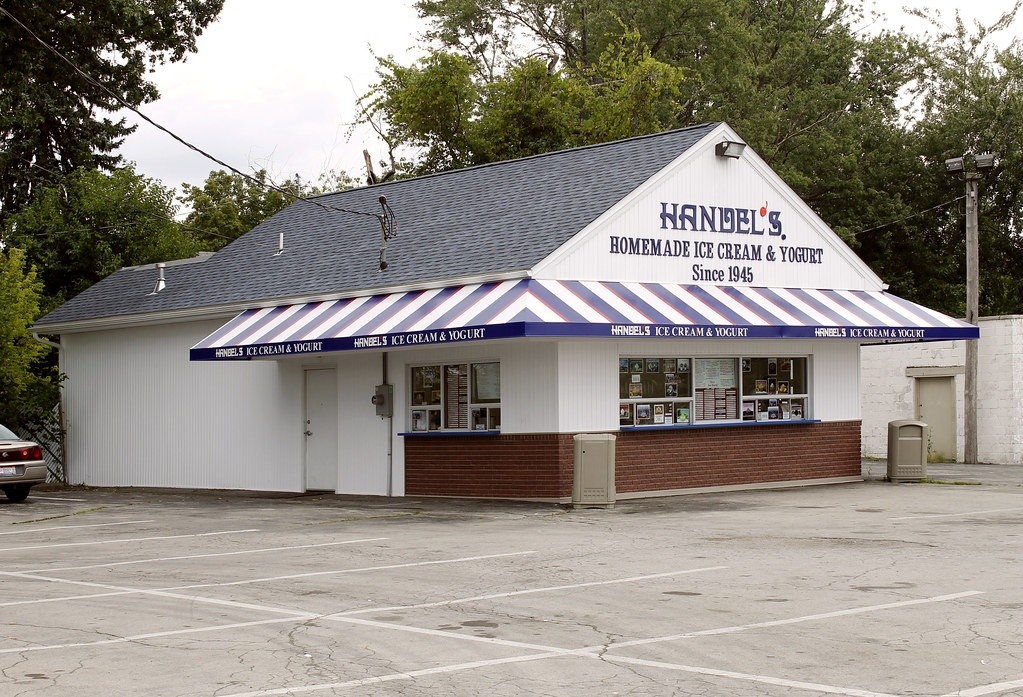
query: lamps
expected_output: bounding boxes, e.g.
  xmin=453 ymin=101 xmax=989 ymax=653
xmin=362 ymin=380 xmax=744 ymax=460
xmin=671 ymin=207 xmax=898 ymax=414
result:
xmin=715 ymin=141 xmax=747 ymax=160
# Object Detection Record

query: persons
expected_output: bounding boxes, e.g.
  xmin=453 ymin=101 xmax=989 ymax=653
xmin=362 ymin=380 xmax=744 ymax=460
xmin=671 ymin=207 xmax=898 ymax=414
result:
xmin=667 ymin=385 xmax=676 ymax=396
xmin=742 ymin=359 xmax=750 ymax=371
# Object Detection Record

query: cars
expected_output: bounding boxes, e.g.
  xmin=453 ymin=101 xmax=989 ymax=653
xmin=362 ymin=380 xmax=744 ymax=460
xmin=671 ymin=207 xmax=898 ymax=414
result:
xmin=0 ymin=423 xmax=47 ymax=504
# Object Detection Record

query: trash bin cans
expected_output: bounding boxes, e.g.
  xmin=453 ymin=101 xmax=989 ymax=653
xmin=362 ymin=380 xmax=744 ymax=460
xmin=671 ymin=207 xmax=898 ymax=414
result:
xmin=886 ymin=419 xmax=928 ymax=483
xmin=571 ymin=433 xmax=617 ymax=510
